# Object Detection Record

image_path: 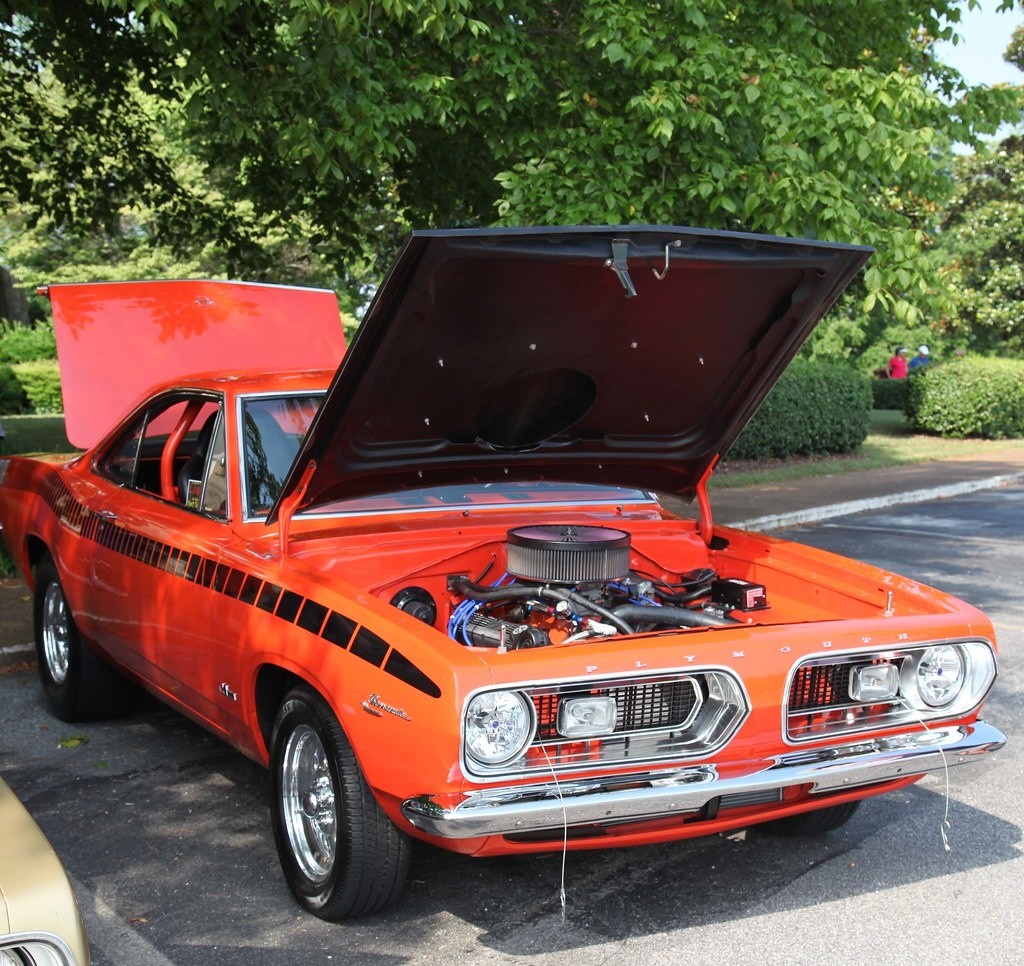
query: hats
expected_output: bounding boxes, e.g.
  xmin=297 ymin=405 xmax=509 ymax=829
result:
xmin=919 ymin=346 xmax=929 ymax=355
xmin=897 ymin=348 xmax=908 ymax=353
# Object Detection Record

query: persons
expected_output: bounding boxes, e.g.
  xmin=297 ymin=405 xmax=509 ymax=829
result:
xmin=908 ymin=344 xmax=932 ymax=371
xmin=885 ymin=346 xmax=908 ymax=380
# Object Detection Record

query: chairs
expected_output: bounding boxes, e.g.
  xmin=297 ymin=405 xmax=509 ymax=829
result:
xmin=180 ymin=404 xmax=297 ymax=518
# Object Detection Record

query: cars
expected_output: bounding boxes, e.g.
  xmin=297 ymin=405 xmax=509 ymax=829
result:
xmin=1 ymin=226 xmax=1008 ymax=923
